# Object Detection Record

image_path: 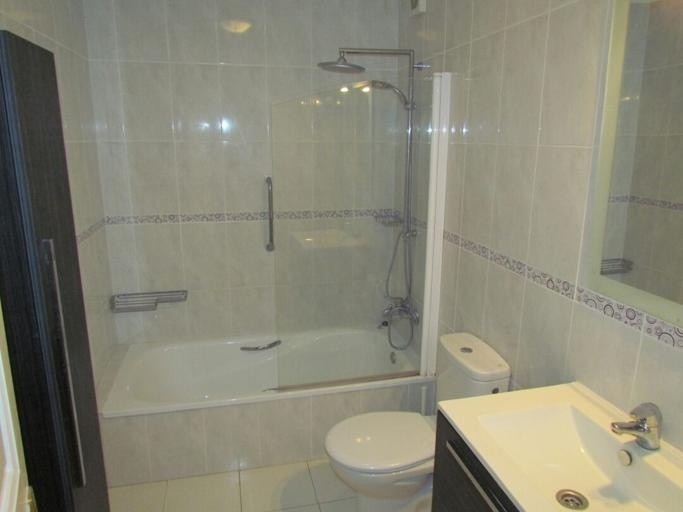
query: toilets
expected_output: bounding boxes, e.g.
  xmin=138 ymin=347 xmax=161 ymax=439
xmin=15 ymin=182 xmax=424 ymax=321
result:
xmin=323 ymin=330 xmax=513 ymax=511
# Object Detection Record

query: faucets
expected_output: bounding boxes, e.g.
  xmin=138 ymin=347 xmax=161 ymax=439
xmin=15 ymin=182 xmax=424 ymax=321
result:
xmin=377 ymin=303 xmax=421 ymax=329
xmin=609 ymin=401 xmax=666 ymax=452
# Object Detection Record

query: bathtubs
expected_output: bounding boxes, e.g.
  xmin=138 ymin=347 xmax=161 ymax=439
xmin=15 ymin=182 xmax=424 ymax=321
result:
xmin=98 ymin=329 xmax=422 ymax=487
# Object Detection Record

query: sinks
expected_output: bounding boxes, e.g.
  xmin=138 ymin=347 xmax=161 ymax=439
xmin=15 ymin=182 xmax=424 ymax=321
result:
xmin=436 ymin=380 xmax=683 ymax=511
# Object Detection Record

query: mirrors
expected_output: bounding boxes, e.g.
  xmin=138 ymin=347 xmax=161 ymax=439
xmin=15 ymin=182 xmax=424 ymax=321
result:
xmin=582 ymin=0 xmax=683 ymax=333
